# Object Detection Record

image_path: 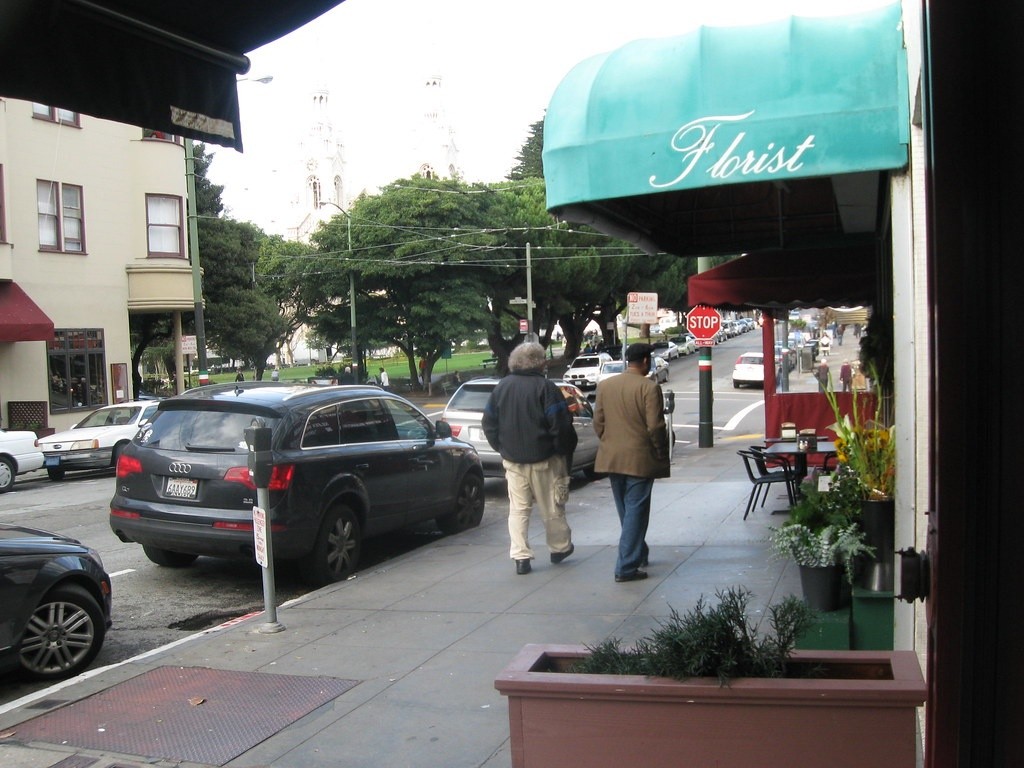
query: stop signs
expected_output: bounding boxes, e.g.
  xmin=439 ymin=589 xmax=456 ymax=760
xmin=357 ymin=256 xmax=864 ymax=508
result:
xmin=686 ymin=305 xmax=721 ymax=340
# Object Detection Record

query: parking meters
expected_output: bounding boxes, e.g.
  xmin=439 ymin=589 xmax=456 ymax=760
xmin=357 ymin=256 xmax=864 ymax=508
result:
xmin=662 ymin=391 xmax=676 ymax=465
xmin=244 ymin=416 xmax=286 ymax=634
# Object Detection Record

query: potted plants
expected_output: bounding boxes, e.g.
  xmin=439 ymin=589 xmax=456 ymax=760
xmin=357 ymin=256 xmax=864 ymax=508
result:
xmin=493 ymin=642 xmax=926 ymax=768
xmin=760 ymin=524 xmax=873 ymax=618
xmin=804 ymin=364 xmax=905 ymax=551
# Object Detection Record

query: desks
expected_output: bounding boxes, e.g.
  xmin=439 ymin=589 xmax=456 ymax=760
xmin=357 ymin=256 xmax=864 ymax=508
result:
xmin=763 ymin=443 xmax=844 ymax=512
xmin=768 ymin=436 xmax=829 ymax=492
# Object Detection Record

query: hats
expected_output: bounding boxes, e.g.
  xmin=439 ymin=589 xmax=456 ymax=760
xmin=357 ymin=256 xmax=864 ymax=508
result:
xmin=821 ymin=359 xmax=827 ymax=364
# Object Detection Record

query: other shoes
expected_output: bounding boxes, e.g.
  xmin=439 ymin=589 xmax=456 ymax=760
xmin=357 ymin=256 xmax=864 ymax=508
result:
xmin=640 ymin=544 xmax=649 ymax=567
xmin=551 ymin=543 xmax=575 ymax=563
xmin=616 ymin=568 xmax=648 ymax=582
xmin=516 ymin=558 xmax=532 ymax=574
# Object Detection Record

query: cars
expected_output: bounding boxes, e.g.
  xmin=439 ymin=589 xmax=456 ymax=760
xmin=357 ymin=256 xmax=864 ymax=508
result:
xmin=0 ymin=522 xmax=114 ymax=680
xmin=0 ymin=429 xmax=44 ymax=493
xmin=597 ymin=317 xmax=817 ymax=390
xmin=37 ymin=400 xmax=160 ymax=481
xmin=440 ymin=379 xmax=608 ymax=480
xmin=562 ymin=352 xmax=613 ymax=391
xmin=732 ymin=352 xmax=781 ymax=391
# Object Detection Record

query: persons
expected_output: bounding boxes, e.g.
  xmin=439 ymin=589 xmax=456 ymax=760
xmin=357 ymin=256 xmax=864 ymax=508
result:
xmin=840 ymin=359 xmax=853 ymax=392
xmin=271 ymin=369 xmax=279 ymax=381
xmin=453 ymin=371 xmax=463 ymax=386
xmin=481 ymin=342 xmax=579 ymax=574
xmin=342 ymin=367 xmax=354 ymax=385
xmin=854 ymin=323 xmax=861 ymax=338
xmin=821 ymin=332 xmax=830 ymax=338
xmin=818 ymin=359 xmax=830 ymax=392
xmin=235 ymin=370 xmax=244 ymax=382
xmin=591 ymin=343 xmax=670 ymax=583
xmin=418 ymin=355 xmax=427 ymax=384
xmin=71 ymin=377 xmax=88 ymax=406
xmin=379 ymin=367 xmax=389 ymax=392
xmin=837 ymin=325 xmax=843 ymax=345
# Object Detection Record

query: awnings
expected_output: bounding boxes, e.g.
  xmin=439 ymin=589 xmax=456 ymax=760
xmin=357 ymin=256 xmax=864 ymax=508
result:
xmin=0 ymin=0 xmax=349 ymax=154
xmin=542 ymin=0 xmax=912 ymax=257
xmin=687 ymin=245 xmax=879 ymax=312
xmin=0 ymin=281 xmax=55 ymax=342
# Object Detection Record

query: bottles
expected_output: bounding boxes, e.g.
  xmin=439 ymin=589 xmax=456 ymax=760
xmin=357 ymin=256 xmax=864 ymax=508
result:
xmin=804 ymin=441 xmax=809 ymax=450
xmin=799 ymin=441 xmax=803 ymax=449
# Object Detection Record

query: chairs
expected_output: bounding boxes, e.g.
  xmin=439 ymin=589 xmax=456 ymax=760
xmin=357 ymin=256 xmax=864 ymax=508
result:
xmin=114 ymin=416 xmax=131 ymax=425
xmin=749 ymin=445 xmax=798 ymax=508
xmin=740 ymin=449 xmax=796 ymax=521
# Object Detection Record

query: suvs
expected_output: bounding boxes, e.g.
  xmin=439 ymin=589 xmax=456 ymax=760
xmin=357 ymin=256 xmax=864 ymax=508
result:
xmin=110 ymin=380 xmax=485 ymax=582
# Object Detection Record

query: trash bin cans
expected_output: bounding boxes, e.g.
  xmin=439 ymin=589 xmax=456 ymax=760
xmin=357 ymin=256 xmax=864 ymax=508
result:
xmin=801 ymin=340 xmax=819 ymax=373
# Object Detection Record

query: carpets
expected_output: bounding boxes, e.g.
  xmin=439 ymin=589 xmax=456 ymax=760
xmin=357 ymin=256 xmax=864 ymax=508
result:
xmin=793 ymin=595 xmax=893 ymax=650
